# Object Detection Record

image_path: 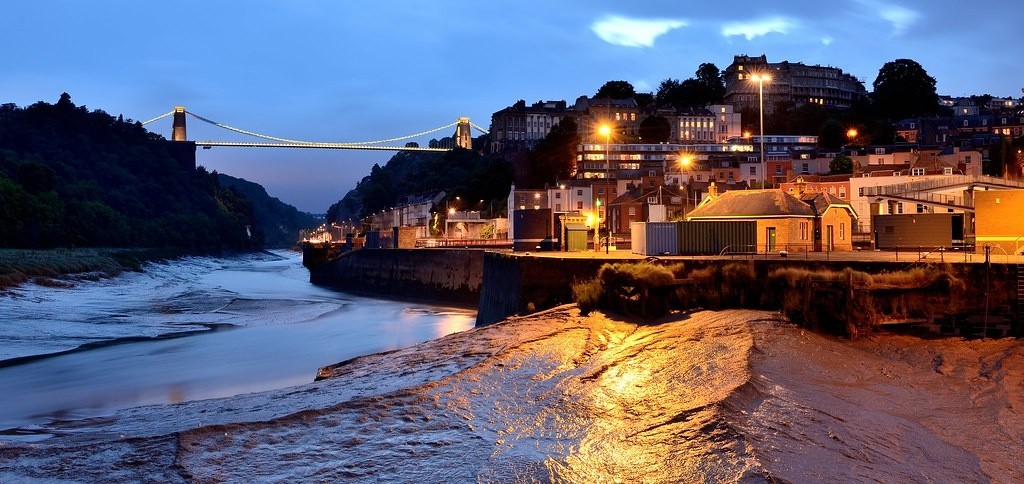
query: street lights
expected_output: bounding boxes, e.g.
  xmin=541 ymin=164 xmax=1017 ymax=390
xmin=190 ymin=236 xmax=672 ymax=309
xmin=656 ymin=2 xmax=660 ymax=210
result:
xmin=596 ymin=122 xmax=611 ymax=254
xmin=679 ymin=154 xmax=695 ymax=221
xmin=297 ymin=217 xmax=352 ymax=242
xmin=750 ymin=74 xmax=772 ymax=189
xmin=848 ymin=128 xmax=859 ymax=156
xmin=456 ymin=196 xmax=468 ymax=219
xmin=479 ymin=199 xmax=494 ymax=239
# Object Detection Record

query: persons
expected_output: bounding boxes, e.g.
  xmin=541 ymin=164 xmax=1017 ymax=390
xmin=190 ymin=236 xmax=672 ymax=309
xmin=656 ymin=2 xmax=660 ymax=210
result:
xmin=325 ymin=240 xmax=332 ymax=248
